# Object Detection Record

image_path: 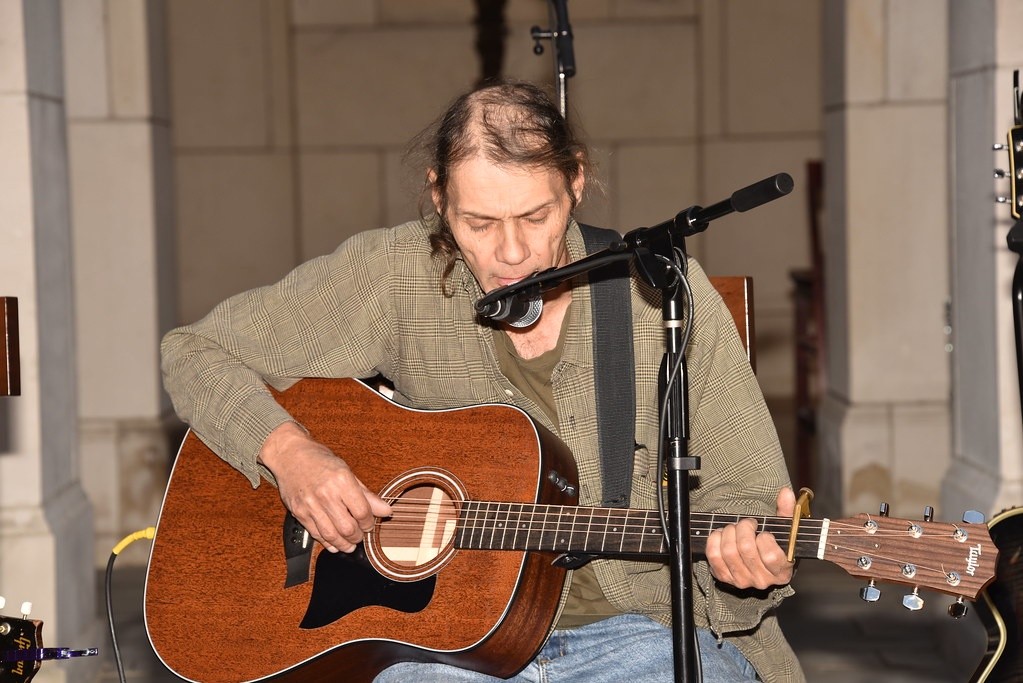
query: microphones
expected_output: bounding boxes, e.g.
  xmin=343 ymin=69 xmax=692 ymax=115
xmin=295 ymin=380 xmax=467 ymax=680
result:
xmin=474 ymin=292 xmax=543 ymax=328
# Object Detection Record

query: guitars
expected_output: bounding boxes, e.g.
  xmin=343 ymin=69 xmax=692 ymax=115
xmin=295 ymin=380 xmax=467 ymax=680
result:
xmin=142 ymin=378 xmax=998 ymax=682
xmin=969 ymin=71 xmax=1023 ymax=680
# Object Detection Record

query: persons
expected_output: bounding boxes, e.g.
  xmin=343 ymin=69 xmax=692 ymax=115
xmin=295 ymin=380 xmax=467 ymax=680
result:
xmin=158 ymin=81 xmax=809 ymax=682
